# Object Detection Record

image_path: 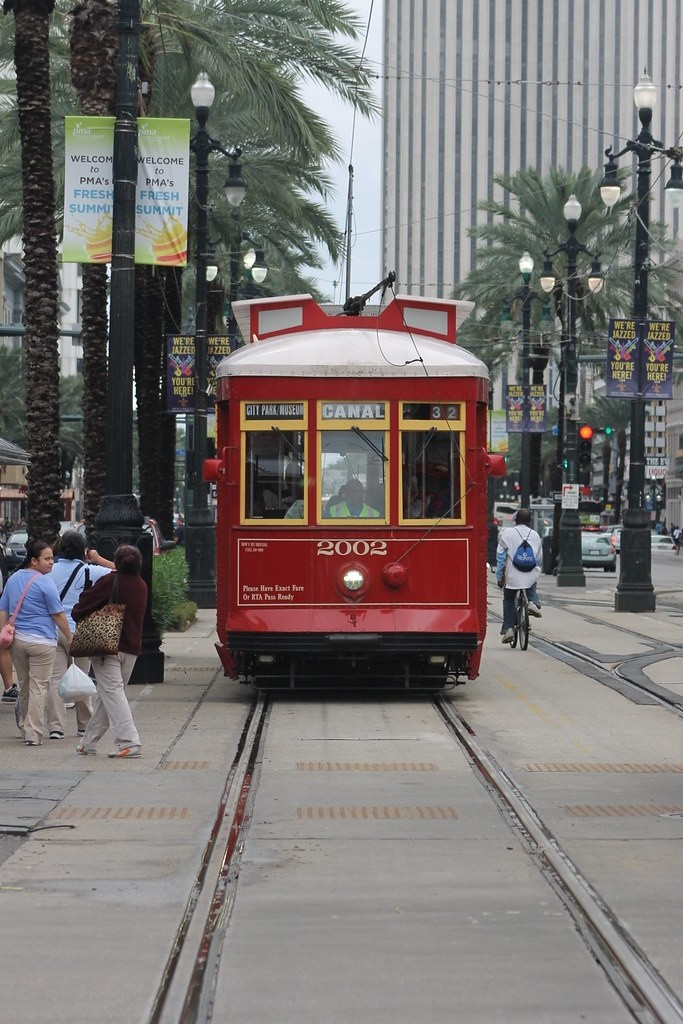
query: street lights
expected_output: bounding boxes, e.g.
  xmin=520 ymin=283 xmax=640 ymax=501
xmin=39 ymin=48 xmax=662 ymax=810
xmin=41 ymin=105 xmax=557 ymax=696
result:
xmin=504 ymin=251 xmax=551 ymax=506
xmin=204 ymin=153 xmax=267 ymax=355
xmin=540 ymin=194 xmax=603 ymax=588
xmin=599 ymin=68 xmax=683 ymax=612
xmin=179 ymin=69 xmax=247 ymax=606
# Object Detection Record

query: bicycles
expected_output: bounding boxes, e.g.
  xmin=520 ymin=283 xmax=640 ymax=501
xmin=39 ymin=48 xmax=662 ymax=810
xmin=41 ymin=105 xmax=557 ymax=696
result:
xmin=509 ymin=589 xmax=530 ymax=648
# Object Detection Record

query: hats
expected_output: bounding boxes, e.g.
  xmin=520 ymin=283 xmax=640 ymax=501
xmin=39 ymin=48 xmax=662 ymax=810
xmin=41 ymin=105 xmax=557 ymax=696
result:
xmin=517 ymin=508 xmax=531 ymax=521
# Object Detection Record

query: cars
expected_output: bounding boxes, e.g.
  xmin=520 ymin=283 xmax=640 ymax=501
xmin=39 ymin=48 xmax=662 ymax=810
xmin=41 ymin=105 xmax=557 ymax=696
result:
xmin=3 ymin=530 xmax=28 ymax=566
xmin=493 ymin=498 xmax=600 ymax=527
xmin=581 ymin=528 xmax=616 ymax=572
xmin=606 ymin=525 xmax=621 ymax=534
xmin=142 ymin=516 xmax=176 ymax=554
xmin=651 ymin=535 xmax=678 ymax=555
xmin=59 ymin=521 xmax=79 ymax=537
xmin=541 ymin=527 xmax=553 ymax=538
xmin=610 ymin=529 xmax=622 ymax=549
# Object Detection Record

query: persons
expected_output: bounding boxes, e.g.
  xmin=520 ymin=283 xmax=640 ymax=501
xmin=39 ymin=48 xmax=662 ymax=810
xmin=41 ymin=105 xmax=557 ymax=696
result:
xmin=324 ymin=478 xmax=384 ymax=519
xmin=486 ymin=514 xmax=499 ymax=573
xmin=497 ymin=508 xmax=543 ymax=643
xmin=174 ymin=518 xmax=185 ymax=545
xmin=282 ymin=463 xmax=303 ymax=498
xmin=0 ymin=530 xmax=115 ymax=745
xmin=655 ymin=519 xmax=683 ymax=550
xmin=71 ymin=544 xmax=146 ymax=757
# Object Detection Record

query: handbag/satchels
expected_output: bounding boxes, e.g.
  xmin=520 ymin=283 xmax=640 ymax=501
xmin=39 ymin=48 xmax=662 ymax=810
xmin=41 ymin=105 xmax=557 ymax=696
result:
xmin=69 ymin=572 xmax=127 ymax=657
xmin=58 ymin=656 xmax=97 ymax=702
xmin=0 ymin=622 xmax=15 ymax=649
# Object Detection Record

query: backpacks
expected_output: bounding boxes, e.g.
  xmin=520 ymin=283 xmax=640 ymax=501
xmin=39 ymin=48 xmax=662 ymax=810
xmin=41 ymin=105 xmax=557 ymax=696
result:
xmin=507 ymin=528 xmax=536 ymax=572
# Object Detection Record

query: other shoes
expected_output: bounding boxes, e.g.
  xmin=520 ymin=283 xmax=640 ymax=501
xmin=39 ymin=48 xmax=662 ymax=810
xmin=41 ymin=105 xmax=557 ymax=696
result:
xmin=65 ymin=703 xmax=75 ymax=708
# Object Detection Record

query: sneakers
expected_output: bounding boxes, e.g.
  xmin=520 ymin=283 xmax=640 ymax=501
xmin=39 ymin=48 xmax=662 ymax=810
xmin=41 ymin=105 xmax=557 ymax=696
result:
xmin=25 ymin=741 xmax=43 ymax=745
xmin=77 ymin=730 xmax=85 ymax=736
xmin=108 ymin=745 xmax=143 ymax=758
xmin=77 ymin=745 xmax=96 ymax=755
xmin=2 ymin=683 xmax=19 ymax=698
xmin=50 ymin=731 xmax=64 ymax=738
xmin=502 ymin=628 xmax=514 ymax=643
xmin=528 ymin=601 xmax=542 ymax=618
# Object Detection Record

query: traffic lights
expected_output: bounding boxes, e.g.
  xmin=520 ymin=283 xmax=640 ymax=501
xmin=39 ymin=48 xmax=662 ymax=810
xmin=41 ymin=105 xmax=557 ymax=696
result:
xmin=592 ymin=427 xmax=615 ymax=435
xmin=577 ymin=422 xmax=592 ymax=483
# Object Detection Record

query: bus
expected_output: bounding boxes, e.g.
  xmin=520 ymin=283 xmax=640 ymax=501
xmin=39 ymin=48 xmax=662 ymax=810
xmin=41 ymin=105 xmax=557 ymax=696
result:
xmin=201 ymin=292 xmax=504 ymax=692
